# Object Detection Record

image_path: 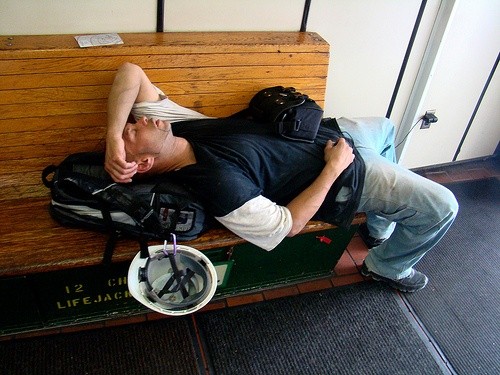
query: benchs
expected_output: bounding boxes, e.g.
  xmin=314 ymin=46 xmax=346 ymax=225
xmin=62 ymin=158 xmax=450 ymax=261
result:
xmin=0 ymin=32 xmax=366 ymax=342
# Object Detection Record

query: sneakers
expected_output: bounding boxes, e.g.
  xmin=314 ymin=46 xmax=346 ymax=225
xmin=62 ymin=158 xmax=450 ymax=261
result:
xmin=360 ymin=260 xmax=428 ymax=293
xmin=362 ymin=220 xmax=387 ymax=249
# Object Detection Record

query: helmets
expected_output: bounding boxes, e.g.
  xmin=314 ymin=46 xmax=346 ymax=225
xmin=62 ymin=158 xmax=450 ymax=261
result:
xmin=128 ymin=244 xmax=218 ymax=316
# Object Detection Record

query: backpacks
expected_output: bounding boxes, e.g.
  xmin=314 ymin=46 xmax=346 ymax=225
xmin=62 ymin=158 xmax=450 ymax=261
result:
xmin=42 ymin=151 xmax=206 ymax=242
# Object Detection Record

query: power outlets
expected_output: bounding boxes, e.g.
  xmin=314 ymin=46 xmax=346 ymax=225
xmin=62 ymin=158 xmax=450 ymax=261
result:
xmin=420 ymin=111 xmax=435 ymax=129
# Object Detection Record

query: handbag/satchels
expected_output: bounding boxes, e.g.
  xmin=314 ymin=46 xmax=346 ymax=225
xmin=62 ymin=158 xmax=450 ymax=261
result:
xmin=251 ymin=85 xmax=324 ymax=142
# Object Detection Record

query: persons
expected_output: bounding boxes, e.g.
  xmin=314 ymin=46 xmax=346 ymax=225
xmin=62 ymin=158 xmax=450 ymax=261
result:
xmin=104 ymin=63 xmax=459 ymax=293
